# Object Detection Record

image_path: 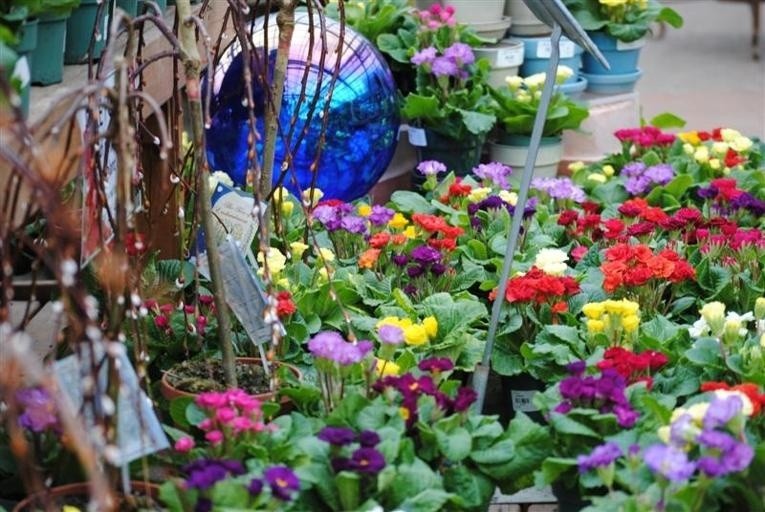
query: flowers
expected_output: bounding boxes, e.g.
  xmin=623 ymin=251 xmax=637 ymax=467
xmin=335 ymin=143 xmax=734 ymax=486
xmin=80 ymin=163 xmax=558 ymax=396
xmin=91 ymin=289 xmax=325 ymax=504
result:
xmin=151 ymin=131 xmax=765 ymax=510
xmin=508 ymin=63 xmax=573 ymax=103
xmin=596 ymin=0 xmax=648 ymax=18
xmin=403 ymin=5 xmax=476 ymax=78
